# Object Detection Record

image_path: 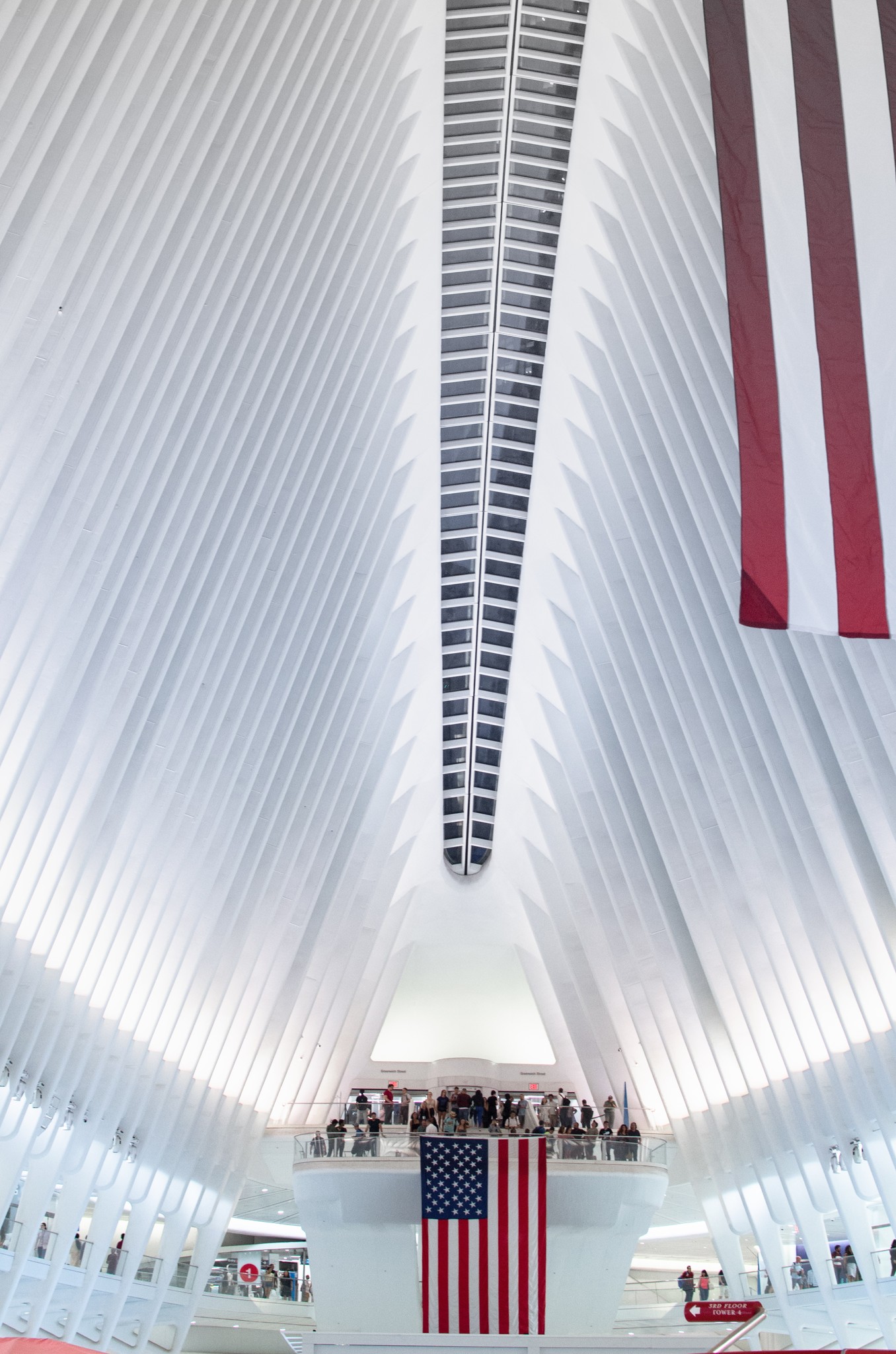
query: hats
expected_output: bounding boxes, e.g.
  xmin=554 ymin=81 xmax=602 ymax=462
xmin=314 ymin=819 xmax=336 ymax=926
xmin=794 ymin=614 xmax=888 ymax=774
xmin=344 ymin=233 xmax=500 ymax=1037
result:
xmin=450 ymin=1111 xmax=457 ymax=1116
xmin=609 ymin=1095 xmax=613 ymax=1099
xmin=593 ymin=1120 xmax=598 ymax=1127
xmin=352 ymin=1123 xmax=359 ymax=1126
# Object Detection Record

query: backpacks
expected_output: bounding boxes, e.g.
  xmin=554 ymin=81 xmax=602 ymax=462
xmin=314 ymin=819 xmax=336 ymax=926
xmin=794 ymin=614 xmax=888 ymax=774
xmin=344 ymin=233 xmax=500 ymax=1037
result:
xmin=559 ymin=1094 xmax=570 ymax=1106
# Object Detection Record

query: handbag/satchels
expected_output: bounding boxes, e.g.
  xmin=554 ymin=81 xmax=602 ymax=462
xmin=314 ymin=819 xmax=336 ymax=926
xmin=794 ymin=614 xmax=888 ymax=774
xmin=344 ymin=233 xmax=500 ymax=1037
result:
xmin=322 ymin=1146 xmax=327 ymax=1156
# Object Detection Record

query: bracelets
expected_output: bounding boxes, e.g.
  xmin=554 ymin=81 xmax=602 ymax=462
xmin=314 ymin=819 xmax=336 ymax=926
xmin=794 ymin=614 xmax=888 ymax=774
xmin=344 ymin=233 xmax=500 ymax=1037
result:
xmin=291 ymin=1286 xmax=293 ymax=1287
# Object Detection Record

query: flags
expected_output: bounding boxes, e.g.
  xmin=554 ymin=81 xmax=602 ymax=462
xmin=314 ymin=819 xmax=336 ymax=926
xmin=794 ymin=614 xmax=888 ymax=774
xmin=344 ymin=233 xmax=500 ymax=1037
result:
xmin=418 ymin=1132 xmax=547 ymax=1335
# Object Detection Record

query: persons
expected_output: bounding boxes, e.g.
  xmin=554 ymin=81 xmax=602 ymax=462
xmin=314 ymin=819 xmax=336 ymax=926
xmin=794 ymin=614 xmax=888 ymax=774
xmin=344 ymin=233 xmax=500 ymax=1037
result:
xmin=889 ymin=1239 xmax=896 ymax=1276
xmin=309 ymin=1119 xmax=366 ymax=1159
xmin=718 ymin=1270 xmax=729 ymax=1300
xmin=0 ymin=1225 xmax=6 ymax=1248
xmin=831 ymin=1245 xmax=863 ymax=1284
xmin=410 ymin=1087 xmax=643 ymax=1163
xmin=698 ymin=1270 xmax=710 ymax=1300
xmin=260 ymin=1264 xmax=279 ymax=1299
xmin=400 ymin=1087 xmax=412 ymax=1124
xmin=34 ymin=1222 xmax=50 ymax=1259
xmin=346 ymin=1084 xmax=403 ymax=1125
xmin=360 ymin=1112 xmax=388 ymax=1157
xmin=106 ymin=1233 xmax=125 ymax=1275
xmin=681 ymin=1265 xmax=694 ymax=1303
xmin=215 ymin=1270 xmax=235 ymax=1295
xmin=800 ymin=1267 xmax=818 ymax=1289
xmin=789 ymin=1255 xmax=805 ymax=1290
xmin=280 ymin=1270 xmax=293 ymax=1300
xmin=70 ymin=1233 xmax=87 ymax=1267
xmin=765 ymin=1268 xmax=774 ymax=1294
xmin=305 ymin=1275 xmax=314 ymax=1302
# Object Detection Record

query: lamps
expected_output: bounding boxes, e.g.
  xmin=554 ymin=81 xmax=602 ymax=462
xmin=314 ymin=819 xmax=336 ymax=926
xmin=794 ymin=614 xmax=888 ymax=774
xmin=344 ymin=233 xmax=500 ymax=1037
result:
xmin=0 ymin=1058 xmax=13 ymax=1087
xmin=109 ymin=1127 xmax=125 ymax=1153
xmin=11 ymin=1070 xmax=30 ymax=1101
xmin=123 ymin=1134 xmax=142 ymax=1162
xmin=63 ymin=1102 xmax=77 ymax=1131
xmin=828 ymin=1147 xmax=847 ymax=1174
xmin=851 ymin=1139 xmax=867 ymax=1164
xmin=30 ymin=1081 xmax=46 ymax=1108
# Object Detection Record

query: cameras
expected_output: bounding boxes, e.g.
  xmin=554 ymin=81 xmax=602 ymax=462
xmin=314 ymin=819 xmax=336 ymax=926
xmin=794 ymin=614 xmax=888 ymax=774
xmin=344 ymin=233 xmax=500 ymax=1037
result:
xmin=610 ymin=1102 xmax=613 ymax=1106
